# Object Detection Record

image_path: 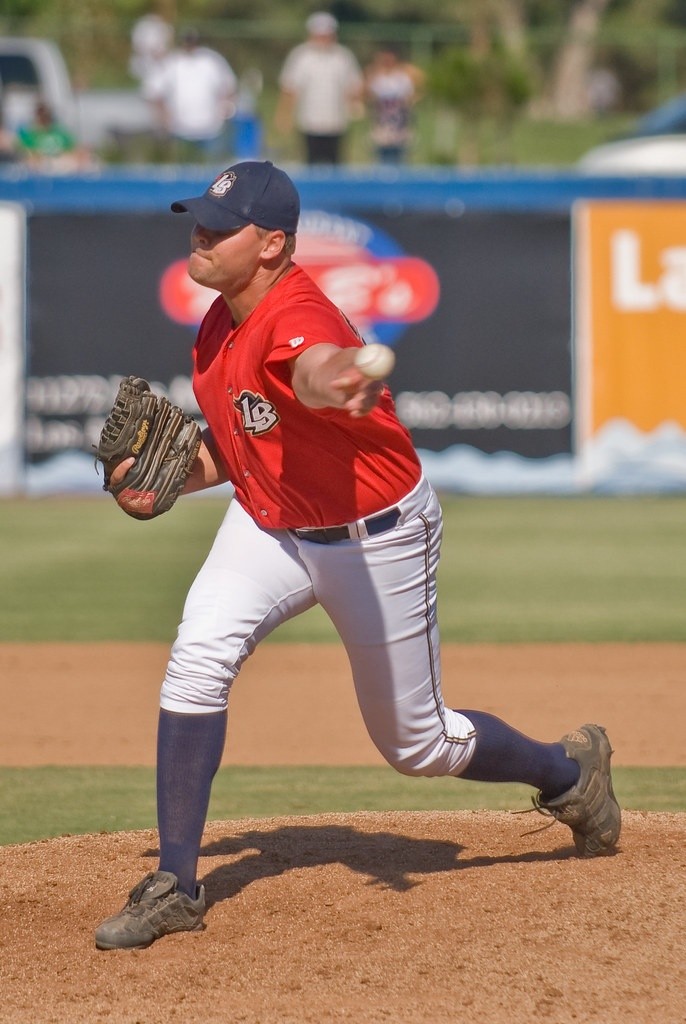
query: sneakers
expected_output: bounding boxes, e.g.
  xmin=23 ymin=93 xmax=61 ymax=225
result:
xmin=95 ymin=871 xmax=206 ymax=950
xmin=510 ymin=723 xmax=622 ymax=858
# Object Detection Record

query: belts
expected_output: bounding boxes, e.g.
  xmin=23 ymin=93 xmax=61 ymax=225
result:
xmin=288 ymin=507 xmax=400 ymax=544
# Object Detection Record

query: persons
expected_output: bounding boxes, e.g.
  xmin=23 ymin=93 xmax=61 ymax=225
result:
xmin=93 ymin=162 xmax=621 ymax=952
xmin=0 ymin=0 xmax=422 ymax=177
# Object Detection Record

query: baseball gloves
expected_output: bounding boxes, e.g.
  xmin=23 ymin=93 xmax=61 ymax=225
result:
xmin=92 ymin=373 xmax=205 ymax=522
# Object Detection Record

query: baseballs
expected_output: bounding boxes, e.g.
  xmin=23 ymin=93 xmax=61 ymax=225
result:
xmin=353 ymin=340 xmax=397 ymax=383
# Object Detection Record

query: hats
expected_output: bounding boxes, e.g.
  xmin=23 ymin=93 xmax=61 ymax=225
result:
xmin=304 ymin=11 xmax=339 ymax=36
xmin=170 ymin=161 xmax=301 ymax=233
